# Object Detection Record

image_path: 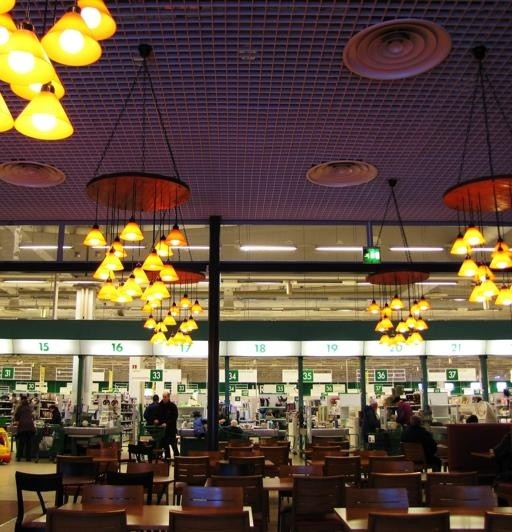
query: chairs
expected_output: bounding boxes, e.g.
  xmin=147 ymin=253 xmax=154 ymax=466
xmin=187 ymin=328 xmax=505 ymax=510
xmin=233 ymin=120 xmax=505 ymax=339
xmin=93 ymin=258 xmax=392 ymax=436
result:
xmin=55 ymin=419 xmax=512 ymax=532
xmin=15 ymin=471 xmax=63 ymax=531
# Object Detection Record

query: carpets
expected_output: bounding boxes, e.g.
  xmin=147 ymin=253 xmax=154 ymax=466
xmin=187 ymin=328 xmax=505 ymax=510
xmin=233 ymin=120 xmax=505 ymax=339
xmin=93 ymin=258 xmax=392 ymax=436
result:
xmin=0 ymin=499 xmax=49 ymax=527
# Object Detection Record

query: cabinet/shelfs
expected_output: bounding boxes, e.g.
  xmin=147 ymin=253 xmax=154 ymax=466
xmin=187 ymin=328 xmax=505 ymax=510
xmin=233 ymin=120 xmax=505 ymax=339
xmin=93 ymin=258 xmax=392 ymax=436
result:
xmin=219 ymin=384 xmax=512 ymax=431
xmin=1 ymin=383 xmax=208 ymax=441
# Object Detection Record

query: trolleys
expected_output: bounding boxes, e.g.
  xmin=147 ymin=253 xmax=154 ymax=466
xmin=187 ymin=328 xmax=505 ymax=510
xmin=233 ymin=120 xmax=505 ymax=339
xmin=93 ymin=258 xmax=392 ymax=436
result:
xmin=367 ymin=429 xmax=402 ymax=457
xmin=135 ymin=421 xmax=167 ymax=450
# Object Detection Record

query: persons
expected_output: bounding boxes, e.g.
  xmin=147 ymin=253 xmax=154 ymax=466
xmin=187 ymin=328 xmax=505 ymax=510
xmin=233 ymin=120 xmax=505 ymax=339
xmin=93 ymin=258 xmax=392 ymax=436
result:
xmin=191 ymin=402 xmax=243 ymax=441
xmin=400 ymin=415 xmax=442 ymax=474
xmin=420 ymin=403 xmax=444 ymax=426
xmin=391 ymin=394 xmax=414 ymax=426
xmin=361 ymin=395 xmax=381 ymax=449
xmin=45 ymin=404 xmax=62 ymax=424
xmin=143 ymin=393 xmax=167 ymax=459
xmin=13 ymin=395 xmax=31 ymax=421
xmin=159 ymin=391 xmax=180 ymax=466
xmin=14 ymin=398 xmax=35 ymax=462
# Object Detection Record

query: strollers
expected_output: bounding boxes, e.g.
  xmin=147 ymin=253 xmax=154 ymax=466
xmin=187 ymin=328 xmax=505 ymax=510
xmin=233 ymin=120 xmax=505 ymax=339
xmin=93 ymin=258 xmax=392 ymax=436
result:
xmin=35 ymin=423 xmax=67 ymax=463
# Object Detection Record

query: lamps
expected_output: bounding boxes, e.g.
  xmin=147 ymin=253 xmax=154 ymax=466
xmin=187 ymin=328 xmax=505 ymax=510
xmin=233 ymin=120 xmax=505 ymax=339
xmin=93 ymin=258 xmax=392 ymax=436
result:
xmin=94 ymin=245 xmax=211 ymax=250
xmin=437 ymin=41 xmax=512 ymax=310
xmin=416 ymin=281 xmax=459 ymax=286
xmin=0 ymin=0 xmax=119 ymax=143
xmin=240 ymin=245 xmax=364 ymax=252
xmin=126 ymin=199 xmax=207 ymax=350
xmin=81 ymin=45 xmax=199 ymax=314
xmin=389 ymin=247 xmax=495 ymax=252
xmin=363 ymin=176 xmax=432 ymax=353
xmin=20 ymin=245 xmax=72 ymax=250
xmin=3 ymin=281 xmax=81 ymax=285
